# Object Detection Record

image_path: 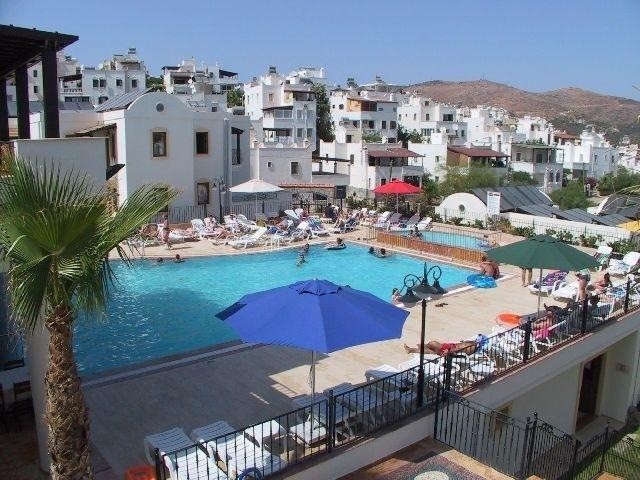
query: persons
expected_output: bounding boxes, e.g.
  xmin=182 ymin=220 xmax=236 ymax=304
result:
xmin=324 ymin=237 xmax=342 ymax=250
xmin=161 ymin=212 xmax=174 ymax=251
xmin=323 ymin=202 xmax=334 ymax=217
xmin=204 ymin=213 xmax=242 ymax=240
xmin=520 ymin=268 xmax=532 ymax=287
xmin=299 ymin=207 xmax=310 ymax=224
xmin=295 ymin=254 xmax=307 ymax=266
xmin=512 ymin=309 xmax=559 ymax=338
xmin=174 ymin=254 xmax=183 ymax=263
xmin=404 ymin=336 xmax=487 ymax=355
xmin=299 ymin=243 xmax=310 ymax=253
xmin=368 ymin=247 xmax=376 ymax=255
xmin=408 ymin=227 xmax=424 ymax=241
xmin=333 ymin=204 xmax=364 ymax=230
xmin=478 ymin=255 xmax=500 ymax=280
xmin=377 ymin=248 xmax=389 ymax=258
xmin=391 ymin=287 xmax=403 ymax=306
xmin=533 ymin=268 xmax=613 ymax=317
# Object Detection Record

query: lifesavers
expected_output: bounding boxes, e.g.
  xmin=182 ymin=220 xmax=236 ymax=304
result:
xmin=467 ymin=273 xmax=495 ymax=288
xmin=495 ymin=314 xmax=520 ymax=326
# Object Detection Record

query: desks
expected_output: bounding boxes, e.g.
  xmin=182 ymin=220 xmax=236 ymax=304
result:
xmin=244 ymin=420 xmax=287 ymax=461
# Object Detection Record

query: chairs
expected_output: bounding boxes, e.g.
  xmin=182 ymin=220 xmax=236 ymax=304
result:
xmin=119 ymin=207 xmax=433 ymax=250
xmin=189 ymin=420 xmax=287 ymax=478
xmin=291 ymin=392 xmax=384 ymax=439
xmin=325 ymin=381 xmax=406 ymax=428
xmin=363 ymin=304 xmax=627 ymax=409
xmin=144 ymin=427 xmax=233 ymax=479
xmin=528 ymin=243 xmax=639 ymax=304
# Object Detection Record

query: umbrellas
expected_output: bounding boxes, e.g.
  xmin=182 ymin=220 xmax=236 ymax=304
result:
xmin=370 ymin=178 xmax=425 ymax=213
xmin=226 ymin=176 xmax=285 ymax=223
xmin=487 ymin=228 xmax=607 ymax=316
xmin=214 ymin=276 xmax=408 ymax=436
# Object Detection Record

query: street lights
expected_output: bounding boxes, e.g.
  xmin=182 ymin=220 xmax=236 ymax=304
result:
xmin=395 ymin=262 xmax=447 ymax=409
